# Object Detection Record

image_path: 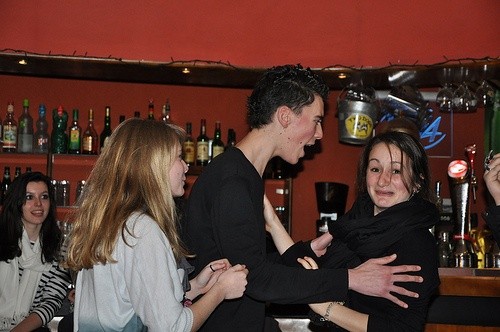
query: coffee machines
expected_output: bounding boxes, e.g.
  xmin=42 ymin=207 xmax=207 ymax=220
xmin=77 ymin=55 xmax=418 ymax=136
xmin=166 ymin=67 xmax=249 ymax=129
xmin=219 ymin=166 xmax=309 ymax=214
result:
xmin=314 ymin=181 xmax=349 ymax=236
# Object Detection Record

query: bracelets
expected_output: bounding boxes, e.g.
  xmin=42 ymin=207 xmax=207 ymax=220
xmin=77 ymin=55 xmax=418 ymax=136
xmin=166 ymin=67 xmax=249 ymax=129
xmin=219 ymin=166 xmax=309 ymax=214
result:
xmin=320 ymin=301 xmax=344 ymax=321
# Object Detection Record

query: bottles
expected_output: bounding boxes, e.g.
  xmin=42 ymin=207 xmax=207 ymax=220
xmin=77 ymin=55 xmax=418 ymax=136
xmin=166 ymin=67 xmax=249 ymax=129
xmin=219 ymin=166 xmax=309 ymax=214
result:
xmin=211 ymin=121 xmax=224 ymax=161
xmin=119 ymin=102 xmax=170 ymax=124
xmin=197 ymin=118 xmax=210 ymax=166
xmin=68 ymin=109 xmax=81 ymax=154
xmin=0 ymin=99 xmax=68 ymax=154
xmin=224 ymin=128 xmax=237 ymax=152
xmin=0 ymin=166 xmax=31 ymax=206
xmin=82 ymin=109 xmax=98 ymax=154
xmin=100 ymin=106 xmax=112 ymax=154
xmin=183 ymin=122 xmax=195 ymax=167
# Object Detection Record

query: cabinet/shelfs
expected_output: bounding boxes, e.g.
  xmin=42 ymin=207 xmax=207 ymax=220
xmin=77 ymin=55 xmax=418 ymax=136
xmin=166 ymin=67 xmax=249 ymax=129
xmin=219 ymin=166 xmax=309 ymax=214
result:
xmin=0 ymin=151 xmax=292 ymax=238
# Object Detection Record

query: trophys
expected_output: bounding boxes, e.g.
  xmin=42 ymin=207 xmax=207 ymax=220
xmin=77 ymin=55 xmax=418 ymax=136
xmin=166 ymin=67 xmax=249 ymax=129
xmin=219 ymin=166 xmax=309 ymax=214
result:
xmin=446 ymin=160 xmax=478 ymax=268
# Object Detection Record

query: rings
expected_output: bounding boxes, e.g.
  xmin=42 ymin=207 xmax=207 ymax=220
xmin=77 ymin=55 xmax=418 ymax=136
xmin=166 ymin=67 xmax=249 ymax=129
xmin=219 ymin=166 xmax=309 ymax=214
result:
xmin=210 ymin=265 xmax=215 ymax=272
xmin=486 ymin=165 xmax=492 ymax=171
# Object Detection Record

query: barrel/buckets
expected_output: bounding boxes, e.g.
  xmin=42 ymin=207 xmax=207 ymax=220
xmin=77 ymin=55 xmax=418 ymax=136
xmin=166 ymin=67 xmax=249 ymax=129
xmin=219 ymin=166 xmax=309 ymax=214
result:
xmin=336 ymin=82 xmax=378 ymax=144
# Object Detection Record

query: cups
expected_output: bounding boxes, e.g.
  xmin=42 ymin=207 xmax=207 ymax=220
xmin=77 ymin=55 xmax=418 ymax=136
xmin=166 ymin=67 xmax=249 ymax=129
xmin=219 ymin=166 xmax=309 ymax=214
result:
xmin=51 ymin=179 xmax=87 ymax=206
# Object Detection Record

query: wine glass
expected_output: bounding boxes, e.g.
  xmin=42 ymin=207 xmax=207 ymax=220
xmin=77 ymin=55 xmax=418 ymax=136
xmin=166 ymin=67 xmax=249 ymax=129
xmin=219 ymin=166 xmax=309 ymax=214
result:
xmin=436 ymin=64 xmax=494 ymax=111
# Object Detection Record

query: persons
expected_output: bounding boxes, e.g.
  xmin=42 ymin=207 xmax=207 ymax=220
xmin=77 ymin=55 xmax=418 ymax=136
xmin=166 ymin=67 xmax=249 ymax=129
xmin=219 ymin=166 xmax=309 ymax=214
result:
xmin=0 ymin=171 xmax=72 ymax=332
xmin=181 ymin=62 xmax=423 ymax=332
xmin=59 ymin=118 xmax=249 ymax=332
xmin=484 ymin=153 xmax=500 ymax=250
xmin=264 ymin=131 xmax=440 ymax=332
